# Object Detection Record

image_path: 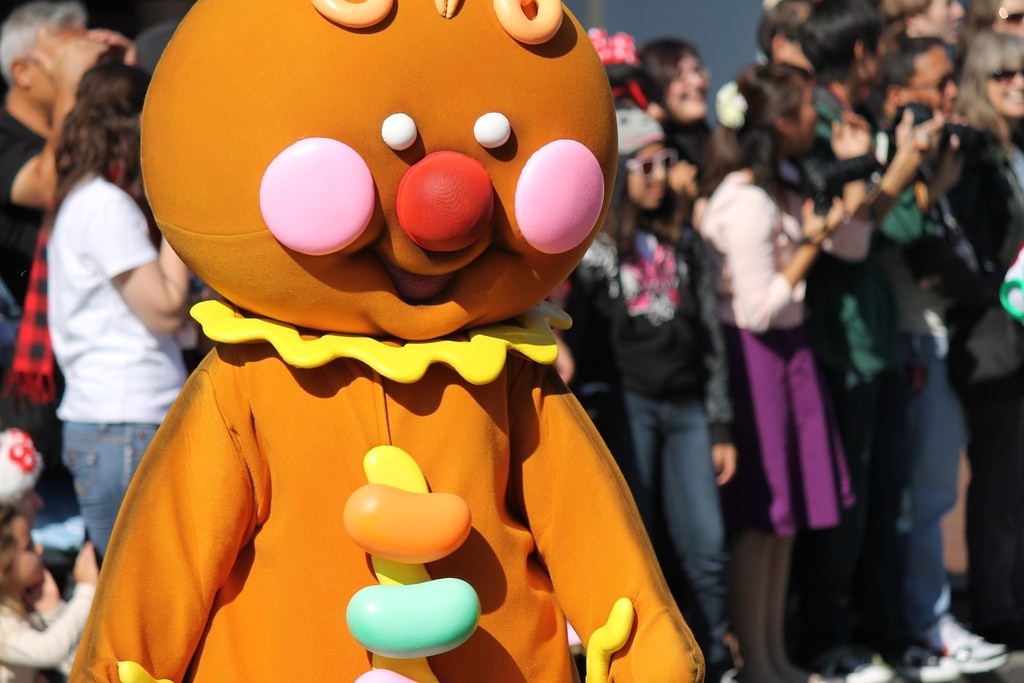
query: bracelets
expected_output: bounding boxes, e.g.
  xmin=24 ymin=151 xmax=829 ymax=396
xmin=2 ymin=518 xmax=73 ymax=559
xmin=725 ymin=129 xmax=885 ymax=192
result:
xmin=799 ymin=236 xmax=820 ymax=246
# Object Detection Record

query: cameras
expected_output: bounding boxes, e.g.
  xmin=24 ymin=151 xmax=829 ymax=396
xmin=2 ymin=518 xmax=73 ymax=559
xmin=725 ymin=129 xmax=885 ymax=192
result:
xmin=802 ymin=156 xmax=875 ymax=209
xmin=891 ymin=103 xmax=990 ymax=156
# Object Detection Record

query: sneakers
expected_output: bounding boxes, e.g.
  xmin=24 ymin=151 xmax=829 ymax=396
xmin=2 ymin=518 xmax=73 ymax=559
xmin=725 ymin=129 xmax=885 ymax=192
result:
xmin=914 ymin=616 xmax=1009 ymax=675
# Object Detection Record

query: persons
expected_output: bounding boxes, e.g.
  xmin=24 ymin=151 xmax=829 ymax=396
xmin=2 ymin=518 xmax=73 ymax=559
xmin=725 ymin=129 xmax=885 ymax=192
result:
xmin=0 ymin=0 xmax=205 ymax=683
xmin=550 ymin=0 xmax=1024 ymax=683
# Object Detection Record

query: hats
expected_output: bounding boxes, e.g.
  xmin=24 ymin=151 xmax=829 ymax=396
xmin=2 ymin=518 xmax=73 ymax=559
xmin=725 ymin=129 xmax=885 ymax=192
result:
xmin=616 ymin=106 xmax=665 ymax=156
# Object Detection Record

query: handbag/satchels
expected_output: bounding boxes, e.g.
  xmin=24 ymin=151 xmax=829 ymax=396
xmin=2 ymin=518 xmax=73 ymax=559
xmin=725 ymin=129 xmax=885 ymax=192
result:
xmin=908 ymin=209 xmax=987 ymax=325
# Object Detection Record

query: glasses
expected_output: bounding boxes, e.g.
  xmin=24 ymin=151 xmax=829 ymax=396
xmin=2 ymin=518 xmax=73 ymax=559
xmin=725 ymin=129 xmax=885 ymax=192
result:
xmin=909 ymin=73 xmax=955 ymax=91
xmin=621 ymin=158 xmax=673 ymax=174
xmin=1005 ymin=12 xmax=1024 ymax=21
xmin=992 ymin=67 xmax=1024 ymax=80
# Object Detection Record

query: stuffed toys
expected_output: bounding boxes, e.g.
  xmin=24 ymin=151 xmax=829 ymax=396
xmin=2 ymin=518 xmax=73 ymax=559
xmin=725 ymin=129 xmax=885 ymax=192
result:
xmin=67 ymin=1 xmax=705 ymax=683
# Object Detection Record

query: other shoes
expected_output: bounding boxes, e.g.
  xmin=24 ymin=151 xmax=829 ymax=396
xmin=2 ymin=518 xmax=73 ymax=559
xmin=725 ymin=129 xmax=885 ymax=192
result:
xmin=816 ymin=647 xmax=893 ymax=683
xmin=895 ymin=646 xmax=960 ymax=679
